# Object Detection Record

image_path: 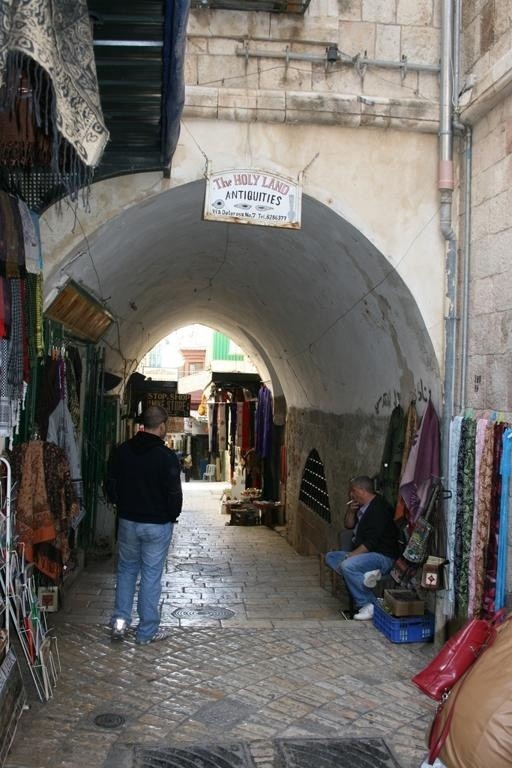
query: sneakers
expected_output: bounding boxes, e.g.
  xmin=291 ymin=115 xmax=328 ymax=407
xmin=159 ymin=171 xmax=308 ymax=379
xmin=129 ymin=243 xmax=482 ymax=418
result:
xmin=363 ymin=569 xmax=382 ymax=589
xmin=135 ymin=629 xmax=169 ymax=645
xmin=112 ymin=617 xmax=129 ymax=642
xmin=353 ymin=602 xmax=375 ymax=622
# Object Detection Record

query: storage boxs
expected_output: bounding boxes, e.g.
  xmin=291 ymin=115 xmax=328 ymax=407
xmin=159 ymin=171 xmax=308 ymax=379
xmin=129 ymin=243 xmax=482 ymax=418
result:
xmin=383 ymin=588 xmax=425 ymax=617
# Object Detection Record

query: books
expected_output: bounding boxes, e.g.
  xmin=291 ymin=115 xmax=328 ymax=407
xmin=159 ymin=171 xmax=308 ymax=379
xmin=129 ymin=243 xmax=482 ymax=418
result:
xmin=8 ymin=554 xmax=58 ymax=702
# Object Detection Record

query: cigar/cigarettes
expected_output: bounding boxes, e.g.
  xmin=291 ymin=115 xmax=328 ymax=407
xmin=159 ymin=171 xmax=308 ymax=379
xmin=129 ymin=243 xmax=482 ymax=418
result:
xmin=347 ymin=500 xmax=353 ymax=505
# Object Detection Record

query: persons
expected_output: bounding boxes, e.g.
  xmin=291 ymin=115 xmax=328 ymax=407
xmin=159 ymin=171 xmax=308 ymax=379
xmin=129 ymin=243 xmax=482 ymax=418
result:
xmin=104 ymin=405 xmax=183 ymax=645
xmin=425 ymin=611 xmax=512 ymax=768
xmin=323 ymin=475 xmax=399 ymax=621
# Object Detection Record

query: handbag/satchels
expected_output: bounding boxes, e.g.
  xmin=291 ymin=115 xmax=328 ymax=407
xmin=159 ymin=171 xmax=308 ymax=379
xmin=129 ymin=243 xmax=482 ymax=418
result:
xmin=409 ymin=607 xmax=506 ymax=765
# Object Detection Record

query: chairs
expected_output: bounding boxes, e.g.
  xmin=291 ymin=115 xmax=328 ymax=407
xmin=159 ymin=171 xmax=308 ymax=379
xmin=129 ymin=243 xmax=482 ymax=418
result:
xmin=204 ymin=464 xmax=216 ymax=482
xmin=318 ymin=529 xmax=356 ymax=596
xmin=349 ymin=529 xmax=403 ymax=620
